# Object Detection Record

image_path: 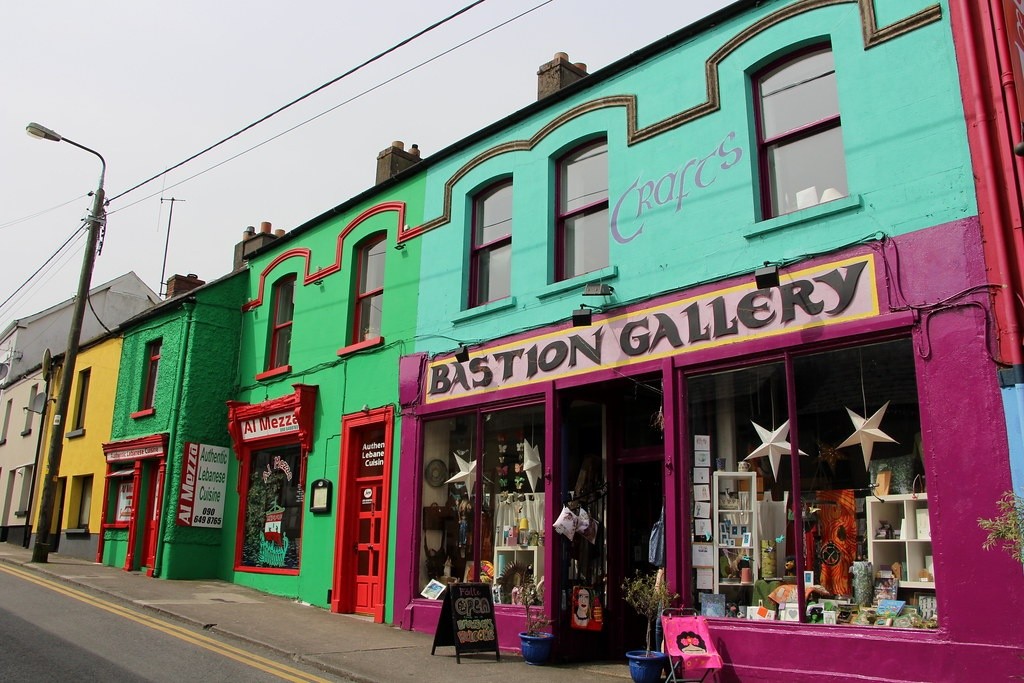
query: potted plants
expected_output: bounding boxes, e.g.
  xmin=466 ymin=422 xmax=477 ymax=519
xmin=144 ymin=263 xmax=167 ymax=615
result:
xmin=518 ymin=566 xmax=554 ymax=666
xmin=620 ymin=567 xmax=680 ymax=683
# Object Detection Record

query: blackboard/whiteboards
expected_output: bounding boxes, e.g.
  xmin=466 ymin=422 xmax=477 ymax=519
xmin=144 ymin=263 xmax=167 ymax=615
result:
xmin=435 ymin=582 xmax=499 ymax=654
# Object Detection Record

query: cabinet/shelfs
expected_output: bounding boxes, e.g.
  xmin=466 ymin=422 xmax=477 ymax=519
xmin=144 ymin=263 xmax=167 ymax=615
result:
xmin=712 ymin=470 xmax=760 ymax=611
xmin=492 ymin=492 xmax=545 ymax=607
xmin=867 ymin=493 xmax=938 ymax=622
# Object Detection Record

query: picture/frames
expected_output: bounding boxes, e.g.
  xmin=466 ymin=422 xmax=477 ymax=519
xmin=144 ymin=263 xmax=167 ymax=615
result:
xmin=571 ymin=586 xmax=603 ymax=632
